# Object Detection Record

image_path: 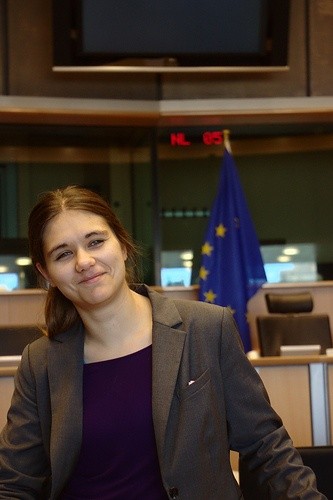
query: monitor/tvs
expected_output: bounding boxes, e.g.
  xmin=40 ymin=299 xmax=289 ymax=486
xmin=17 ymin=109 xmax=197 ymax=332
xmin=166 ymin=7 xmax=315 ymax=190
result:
xmin=258 ymin=243 xmax=318 ymax=284
xmin=51 ymin=0 xmax=290 ymax=72
xmin=0 ymin=255 xmax=26 ymax=290
xmin=160 ymin=249 xmax=195 ymax=287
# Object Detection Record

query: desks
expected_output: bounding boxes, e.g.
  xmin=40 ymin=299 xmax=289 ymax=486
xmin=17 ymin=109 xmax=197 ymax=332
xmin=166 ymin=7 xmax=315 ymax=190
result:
xmin=0 ymin=354 xmax=333 ymax=472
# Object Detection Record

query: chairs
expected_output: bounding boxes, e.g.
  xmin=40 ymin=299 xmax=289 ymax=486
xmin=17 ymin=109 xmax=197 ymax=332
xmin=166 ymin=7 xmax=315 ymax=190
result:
xmin=255 ymin=292 xmax=333 ymax=357
xmin=0 ymin=325 xmax=44 ymax=356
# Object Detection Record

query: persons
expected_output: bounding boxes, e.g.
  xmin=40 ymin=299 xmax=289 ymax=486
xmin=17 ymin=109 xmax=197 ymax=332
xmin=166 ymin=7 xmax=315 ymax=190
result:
xmin=0 ymin=184 xmax=330 ymax=500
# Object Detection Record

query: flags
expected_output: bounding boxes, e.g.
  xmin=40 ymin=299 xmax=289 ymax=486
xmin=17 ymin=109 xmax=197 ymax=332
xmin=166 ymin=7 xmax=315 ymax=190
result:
xmin=199 ymin=142 xmax=267 ymax=354
